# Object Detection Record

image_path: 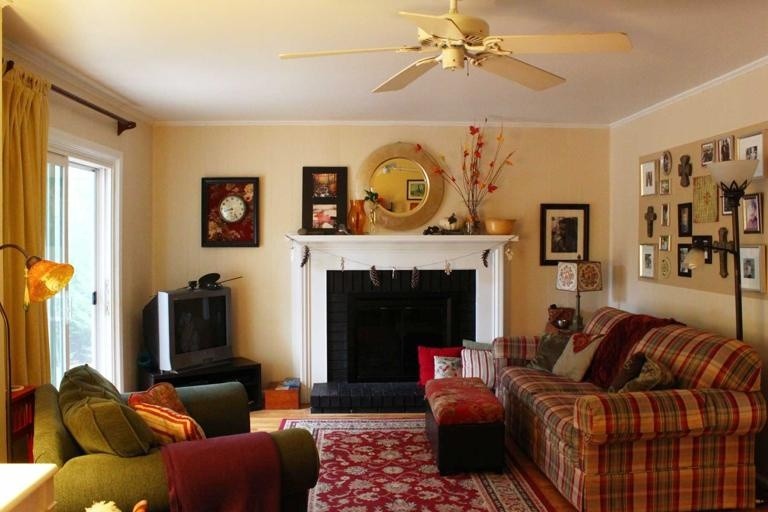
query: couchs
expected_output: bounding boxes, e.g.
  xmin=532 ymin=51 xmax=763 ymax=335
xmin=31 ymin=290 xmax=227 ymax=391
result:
xmin=35 ymin=380 xmax=320 ymax=512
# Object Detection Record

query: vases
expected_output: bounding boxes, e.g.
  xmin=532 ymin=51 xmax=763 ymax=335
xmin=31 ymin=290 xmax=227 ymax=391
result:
xmin=368 ymin=206 xmax=377 ymax=234
xmin=464 ymin=201 xmax=480 ymax=234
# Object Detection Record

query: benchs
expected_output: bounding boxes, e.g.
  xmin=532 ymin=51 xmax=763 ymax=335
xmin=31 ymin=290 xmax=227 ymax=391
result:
xmin=425 ymin=377 xmax=508 ymax=474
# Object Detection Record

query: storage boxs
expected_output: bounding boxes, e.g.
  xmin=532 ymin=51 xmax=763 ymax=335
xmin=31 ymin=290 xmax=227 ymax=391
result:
xmin=263 ymin=381 xmax=300 ymax=410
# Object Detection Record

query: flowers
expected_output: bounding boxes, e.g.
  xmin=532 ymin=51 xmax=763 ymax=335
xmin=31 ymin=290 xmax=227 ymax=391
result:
xmin=416 ymin=119 xmax=515 ymax=227
xmin=364 ymin=189 xmax=384 ymax=224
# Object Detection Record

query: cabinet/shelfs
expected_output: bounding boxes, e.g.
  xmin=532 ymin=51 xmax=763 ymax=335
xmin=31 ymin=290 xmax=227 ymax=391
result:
xmin=139 ymin=356 xmax=262 ymax=410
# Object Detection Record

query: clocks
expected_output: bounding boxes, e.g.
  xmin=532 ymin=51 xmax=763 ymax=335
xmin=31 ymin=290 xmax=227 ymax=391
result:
xmin=220 ymin=196 xmax=247 ymax=224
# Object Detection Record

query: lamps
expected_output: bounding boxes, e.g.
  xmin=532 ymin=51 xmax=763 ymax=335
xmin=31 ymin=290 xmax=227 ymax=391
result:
xmin=0 ymin=244 xmax=74 ymax=462
xmin=707 ymin=160 xmax=757 ymax=341
xmin=555 ymin=254 xmax=603 ymax=330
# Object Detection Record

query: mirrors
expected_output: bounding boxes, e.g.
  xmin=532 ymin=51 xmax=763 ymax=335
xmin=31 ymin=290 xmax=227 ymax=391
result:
xmin=355 ymin=142 xmax=444 ymax=233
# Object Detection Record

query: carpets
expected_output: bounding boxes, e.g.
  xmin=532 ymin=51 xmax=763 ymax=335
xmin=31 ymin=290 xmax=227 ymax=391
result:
xmin=280 ymin=416 xmax=556 ymax=512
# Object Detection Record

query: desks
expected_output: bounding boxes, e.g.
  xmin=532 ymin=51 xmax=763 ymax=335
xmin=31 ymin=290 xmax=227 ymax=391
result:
xmin=12 ymin=384 xmax=37 ymax=463
xmin=0 ymin=464 xmax=58 ymax=512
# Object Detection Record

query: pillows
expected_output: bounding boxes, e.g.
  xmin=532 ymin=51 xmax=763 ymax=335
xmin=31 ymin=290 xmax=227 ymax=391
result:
xmin=418 ymin=341 xmax=495 ymax=388
xmin=530 ymin=332 xmax=676 ymax=393
xmin=59 ymin=363 xmax=205 ymax=458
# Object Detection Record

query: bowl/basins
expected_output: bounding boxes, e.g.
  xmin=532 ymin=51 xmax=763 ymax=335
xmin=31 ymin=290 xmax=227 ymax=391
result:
xmin=483 ymin=217 xmax=517 ymax=234
xmin=548 ymin=314 xmax=582 ymax=332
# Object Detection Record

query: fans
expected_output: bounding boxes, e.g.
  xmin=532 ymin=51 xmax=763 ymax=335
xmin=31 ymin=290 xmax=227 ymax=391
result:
xmin=278 ymin=0 xmax=634 ymax=92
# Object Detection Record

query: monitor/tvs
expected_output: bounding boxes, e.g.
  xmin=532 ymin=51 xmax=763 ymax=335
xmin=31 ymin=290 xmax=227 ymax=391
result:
xmin=140 ymin=284 xmax=232 ymax=375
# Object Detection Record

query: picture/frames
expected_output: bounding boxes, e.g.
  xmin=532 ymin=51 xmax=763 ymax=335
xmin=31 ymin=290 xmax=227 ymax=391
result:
xmin=540 ymin=203 xmax=590 ymax=266
xmin=201 ymin=177 xmax=259 ymax=247
xmin=302 ymin=166 xmax=348 ymax=235
xmin=407 ymin=179 xmax=425 ymax=200
xmin=640 ymin=121 xmax=767 ymax=293
